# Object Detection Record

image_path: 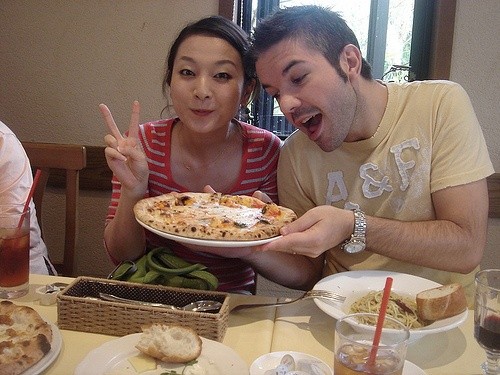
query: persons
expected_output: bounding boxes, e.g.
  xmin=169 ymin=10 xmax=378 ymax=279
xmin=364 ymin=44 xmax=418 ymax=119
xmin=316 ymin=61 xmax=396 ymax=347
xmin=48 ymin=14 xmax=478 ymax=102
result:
xmin=246 ymin=5 xmax=495 ymax=312
xmin=0 ymin=118 xmax=59 ymax=277
xmin=98 ymin=15 xmax=286 ymax=295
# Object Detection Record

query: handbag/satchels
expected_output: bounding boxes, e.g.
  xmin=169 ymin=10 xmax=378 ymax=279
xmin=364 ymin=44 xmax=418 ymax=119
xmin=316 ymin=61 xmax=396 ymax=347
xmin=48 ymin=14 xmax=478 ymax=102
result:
xmin=107 ymin=246 xmax=219 ymax=291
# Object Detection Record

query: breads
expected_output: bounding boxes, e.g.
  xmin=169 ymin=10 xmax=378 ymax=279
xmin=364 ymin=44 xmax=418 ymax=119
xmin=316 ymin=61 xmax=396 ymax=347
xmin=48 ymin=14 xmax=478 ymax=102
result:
xmin=416 ymin=281 xmax=466 ymax=320
xmin=135 ymin=323 xmax=202 ymax=363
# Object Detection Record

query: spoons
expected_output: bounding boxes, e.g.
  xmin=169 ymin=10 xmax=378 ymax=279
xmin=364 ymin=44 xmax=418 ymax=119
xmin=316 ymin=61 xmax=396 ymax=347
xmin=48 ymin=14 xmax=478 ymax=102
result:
xmin=100 ymin=289 xmax=223 ymax=312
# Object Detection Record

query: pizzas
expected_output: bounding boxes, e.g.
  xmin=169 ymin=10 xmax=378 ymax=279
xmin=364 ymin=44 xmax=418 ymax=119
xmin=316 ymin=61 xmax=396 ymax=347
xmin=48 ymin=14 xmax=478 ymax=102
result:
xmin=134 ymin=191 xmax=298 ymax=241
xmin=0 ymin=301 xmax=52 ymax=374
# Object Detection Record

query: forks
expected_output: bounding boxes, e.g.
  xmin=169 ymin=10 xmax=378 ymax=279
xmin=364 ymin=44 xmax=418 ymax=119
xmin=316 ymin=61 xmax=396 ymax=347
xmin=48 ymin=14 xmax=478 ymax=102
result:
xmin=229 ymin=289 xmax=347 ymax=316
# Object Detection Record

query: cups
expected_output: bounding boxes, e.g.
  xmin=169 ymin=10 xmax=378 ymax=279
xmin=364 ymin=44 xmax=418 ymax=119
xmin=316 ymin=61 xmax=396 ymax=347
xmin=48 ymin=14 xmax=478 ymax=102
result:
xmin=334 ymin=313 xmax=410 ymax=375
xmin=0 ymin=203 xmax=30 ymax=300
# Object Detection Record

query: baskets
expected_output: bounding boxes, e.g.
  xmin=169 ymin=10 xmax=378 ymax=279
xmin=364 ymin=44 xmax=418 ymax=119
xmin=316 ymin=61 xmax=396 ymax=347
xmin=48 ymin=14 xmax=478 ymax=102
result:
xmin=57 ymin=276 xmax=230 ymax=342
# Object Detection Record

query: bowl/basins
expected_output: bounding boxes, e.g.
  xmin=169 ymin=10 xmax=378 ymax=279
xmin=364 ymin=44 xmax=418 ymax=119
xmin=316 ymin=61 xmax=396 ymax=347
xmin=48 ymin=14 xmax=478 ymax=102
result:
xmin=35 ymin=285 xmax=60 ymax=306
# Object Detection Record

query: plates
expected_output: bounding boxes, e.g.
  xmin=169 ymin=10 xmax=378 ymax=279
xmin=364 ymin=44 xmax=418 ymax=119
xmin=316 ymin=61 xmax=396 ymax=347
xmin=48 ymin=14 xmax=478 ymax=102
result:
xmin=0 ymin=321 xmax=62 ymax=375
xmin=135 ymin=215 xmax=285 ymax=249
xmin=312 ymin=269 xmax=469 ymax=343
xmin=74 ymin=332 xmax=248 ymax=375
xmin=250 ymin=351 xmax=333 ymax=375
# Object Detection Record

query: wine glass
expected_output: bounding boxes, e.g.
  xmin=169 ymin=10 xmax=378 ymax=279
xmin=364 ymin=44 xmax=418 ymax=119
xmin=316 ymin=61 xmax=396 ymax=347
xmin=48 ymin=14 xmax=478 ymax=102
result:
xmin=473 ymin=268 xmax=500 ymax=375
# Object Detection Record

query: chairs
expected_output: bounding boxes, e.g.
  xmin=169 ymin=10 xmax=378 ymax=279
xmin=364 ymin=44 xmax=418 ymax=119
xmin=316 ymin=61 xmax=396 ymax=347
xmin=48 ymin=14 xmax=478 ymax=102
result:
xmin=19 ymin=141 xmax=86 ymax=276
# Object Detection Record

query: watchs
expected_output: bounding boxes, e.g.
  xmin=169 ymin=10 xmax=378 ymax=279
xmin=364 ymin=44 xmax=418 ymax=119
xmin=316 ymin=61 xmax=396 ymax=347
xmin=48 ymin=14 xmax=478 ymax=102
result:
xmin=341 ymin=208 xmax=368 ymax=255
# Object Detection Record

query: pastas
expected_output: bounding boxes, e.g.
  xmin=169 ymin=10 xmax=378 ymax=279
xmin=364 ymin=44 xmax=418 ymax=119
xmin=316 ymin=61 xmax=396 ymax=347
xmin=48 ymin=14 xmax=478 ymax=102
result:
xmin=352 ymin=287 xmax=433 ymax=331
